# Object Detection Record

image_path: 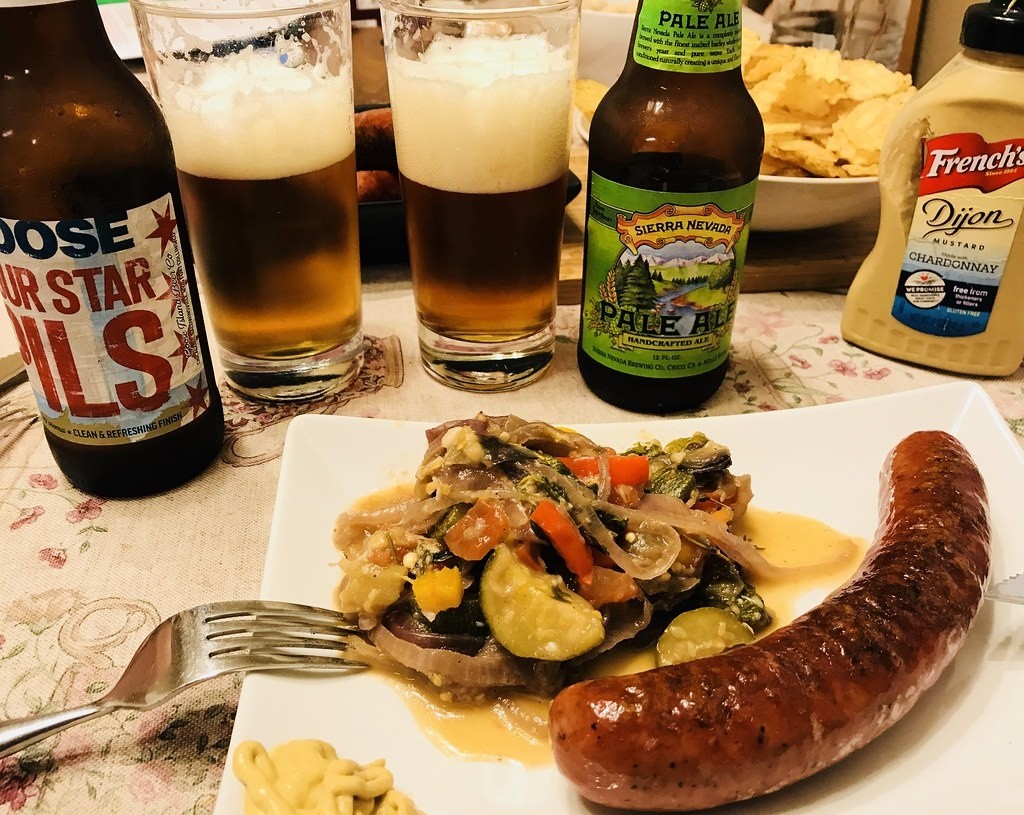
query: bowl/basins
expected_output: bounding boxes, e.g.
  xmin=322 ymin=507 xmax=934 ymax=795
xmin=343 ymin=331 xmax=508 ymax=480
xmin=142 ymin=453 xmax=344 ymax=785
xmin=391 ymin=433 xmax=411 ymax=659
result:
xmin=752 ymin=172 xmax=883 ymax=233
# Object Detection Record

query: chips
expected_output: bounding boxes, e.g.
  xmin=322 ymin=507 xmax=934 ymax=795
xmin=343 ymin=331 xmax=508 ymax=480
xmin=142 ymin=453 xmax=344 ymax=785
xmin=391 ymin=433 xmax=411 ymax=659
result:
xmin=741 ymin=30 xmax=919 ymax=180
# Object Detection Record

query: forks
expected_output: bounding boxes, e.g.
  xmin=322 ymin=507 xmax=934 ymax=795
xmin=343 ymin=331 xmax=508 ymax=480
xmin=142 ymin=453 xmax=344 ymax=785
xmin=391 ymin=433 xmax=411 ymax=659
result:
xmin=0 ymin=595 xmax=372 ymax=757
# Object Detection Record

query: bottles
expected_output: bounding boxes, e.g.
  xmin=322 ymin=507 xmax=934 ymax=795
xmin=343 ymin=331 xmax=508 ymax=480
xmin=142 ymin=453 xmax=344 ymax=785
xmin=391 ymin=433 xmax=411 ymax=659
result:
xmin=580 ymin=0 xmax=770 ymax=415
xmin=2 ymin=1 xmax=225 ymax=502
xmin=837 ymin=1 xmax=1024 ymax=381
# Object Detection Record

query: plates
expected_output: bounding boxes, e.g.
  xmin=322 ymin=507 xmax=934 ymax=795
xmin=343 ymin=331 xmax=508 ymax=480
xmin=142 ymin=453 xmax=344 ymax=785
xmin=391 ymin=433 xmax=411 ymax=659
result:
xmin=208 ymin=380 xmax=1023 ymax=815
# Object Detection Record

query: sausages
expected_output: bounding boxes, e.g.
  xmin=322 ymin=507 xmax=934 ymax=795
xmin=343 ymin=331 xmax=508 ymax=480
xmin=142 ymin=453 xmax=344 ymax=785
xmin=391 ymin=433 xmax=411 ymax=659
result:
xmin=550 ymin=430 xmax=993 ymax=814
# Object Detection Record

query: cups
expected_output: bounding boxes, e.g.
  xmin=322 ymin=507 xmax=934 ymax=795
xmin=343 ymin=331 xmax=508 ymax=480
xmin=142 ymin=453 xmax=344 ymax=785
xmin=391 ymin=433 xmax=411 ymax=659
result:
xmin=377 ymin=0 xmax=591 ymax=397
xmin=128 ymin=0 xmax=365 ymax=402
